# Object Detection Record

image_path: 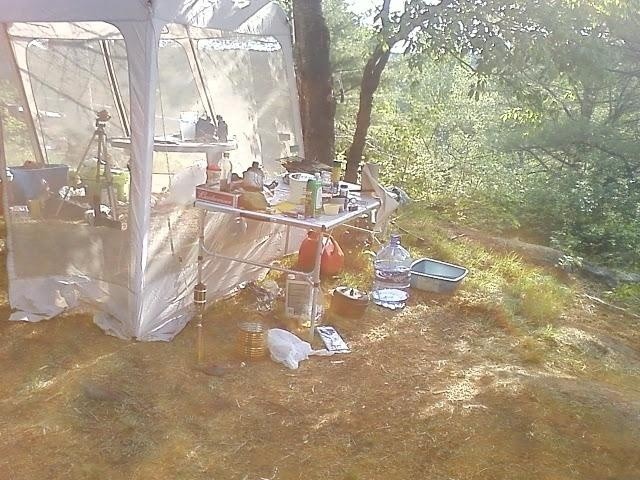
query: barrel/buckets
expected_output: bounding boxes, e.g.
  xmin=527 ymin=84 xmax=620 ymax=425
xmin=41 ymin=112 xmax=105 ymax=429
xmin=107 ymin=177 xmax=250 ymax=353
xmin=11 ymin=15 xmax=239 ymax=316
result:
xmin=237 ymin=322 xmax=266 ymax=359
xmin=285 ymin=276 xmax=311 ymax=319
xmin=370 ymin=235 xmax=412 ymax=310
xmin=298 ymin=231 xmax=345 ymax=275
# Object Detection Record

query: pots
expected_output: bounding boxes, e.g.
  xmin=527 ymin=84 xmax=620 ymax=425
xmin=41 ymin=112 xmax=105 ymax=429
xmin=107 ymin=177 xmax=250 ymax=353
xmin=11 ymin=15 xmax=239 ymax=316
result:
xmin=332 ymin=285 xmax=369 ymax=319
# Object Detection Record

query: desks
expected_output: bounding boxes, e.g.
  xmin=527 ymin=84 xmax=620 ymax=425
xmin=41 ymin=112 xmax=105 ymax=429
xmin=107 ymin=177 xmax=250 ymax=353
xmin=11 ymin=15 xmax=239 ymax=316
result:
xmin=112 ymin=136 xmax=238 ymax=254
xmin=195 ymin=171 xmax=398 ymax=364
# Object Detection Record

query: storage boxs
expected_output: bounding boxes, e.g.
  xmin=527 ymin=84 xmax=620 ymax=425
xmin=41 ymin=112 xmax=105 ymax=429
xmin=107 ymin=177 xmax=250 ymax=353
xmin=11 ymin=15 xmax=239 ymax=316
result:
xmin=7 ymin=163 xmax=69 ymax=201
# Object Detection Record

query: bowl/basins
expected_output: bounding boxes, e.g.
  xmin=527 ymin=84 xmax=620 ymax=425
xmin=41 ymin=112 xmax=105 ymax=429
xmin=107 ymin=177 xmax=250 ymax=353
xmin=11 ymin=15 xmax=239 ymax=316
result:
xmin=324 ymin=204 xmax=339 ymax=214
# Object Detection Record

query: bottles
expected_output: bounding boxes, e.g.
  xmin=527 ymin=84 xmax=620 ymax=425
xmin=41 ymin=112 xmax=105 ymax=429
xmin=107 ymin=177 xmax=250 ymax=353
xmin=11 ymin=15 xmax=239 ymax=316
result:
xmin=204 ymin=164 xmax=220 ymax=191
xmin=304 ymin=178 xmax=322 ymax=216
xmin=340 ymin=184 xmax=348 ymax=211
xmin=332 ymin=160 xmax=341 ymax=183
xmin=219 ymin=152 xmax=232 ymax=194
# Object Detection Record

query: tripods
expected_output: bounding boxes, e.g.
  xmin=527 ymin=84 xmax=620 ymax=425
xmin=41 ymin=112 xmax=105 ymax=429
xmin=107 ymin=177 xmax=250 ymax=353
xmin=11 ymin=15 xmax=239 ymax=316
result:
xmin=55 ymin=129 xmax=119 ymax=228
